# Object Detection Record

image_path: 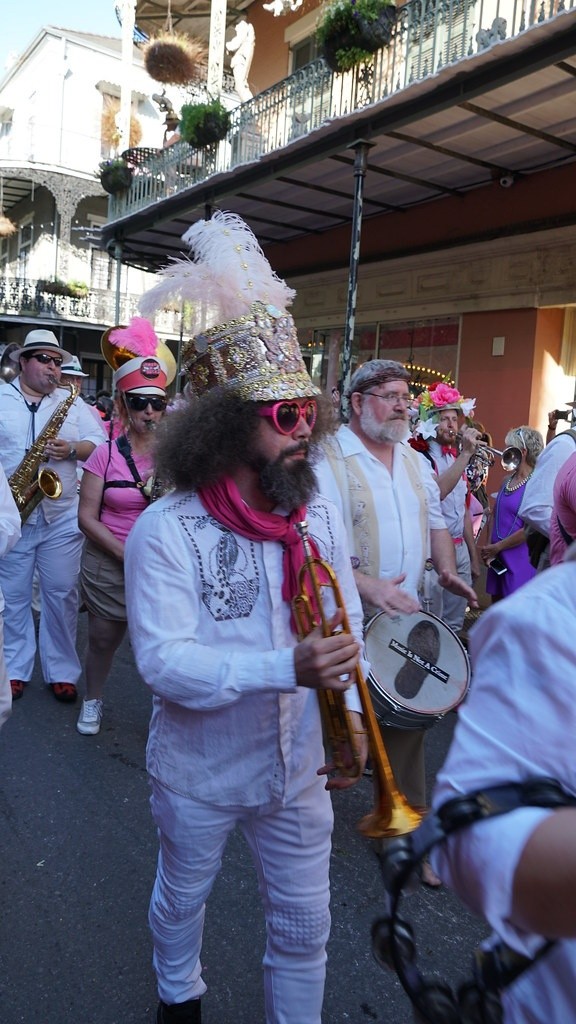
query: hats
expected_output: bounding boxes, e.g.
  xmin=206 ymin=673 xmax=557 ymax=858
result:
xmin=8 ymin=329 xmax=73 ymax=367
xmin=100 ymin=323 xmax=177 ymax=395
xmin=410 ymin=390 xmax=476 ymax=418
xmin=137 ymin=211 xmax=322 ymax=400
xmin=341 ymin=360 xmax=412 ymax=418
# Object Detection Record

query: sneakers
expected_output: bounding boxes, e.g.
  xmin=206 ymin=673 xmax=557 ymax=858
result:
xmin=76 ymin=696 xmax=104 ymax=735
xmin=10 ymin=679 xmax=25 ymax=700
xmin=49 ymin=682 xmax=77 ymax=702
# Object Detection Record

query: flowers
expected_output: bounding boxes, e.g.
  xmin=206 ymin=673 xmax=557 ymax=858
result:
xmin=312 ymin=0 xmax=396 ymax=45
xmin=93 ymin=160 xmax=128 ymax=179
xmin=408 ymin=370 xmax=477 ymax=441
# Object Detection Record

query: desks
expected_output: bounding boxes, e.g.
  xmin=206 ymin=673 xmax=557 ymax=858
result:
xmin=122 ymin=147 xmax=160 ymax=165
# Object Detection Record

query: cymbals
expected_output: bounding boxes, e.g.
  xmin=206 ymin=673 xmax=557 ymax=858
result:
xmin=372 ymin=777 xmax=576 ymax=1024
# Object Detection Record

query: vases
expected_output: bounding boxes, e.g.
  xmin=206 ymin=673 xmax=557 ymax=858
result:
xmin=102 ymin=166 xmax=131 ymax=193
xmin=323 ymin=6 xmax=398 ymax=73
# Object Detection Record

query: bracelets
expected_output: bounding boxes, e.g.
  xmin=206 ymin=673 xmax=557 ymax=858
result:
xmin=548 ymin=424 xmax=556 ymax=430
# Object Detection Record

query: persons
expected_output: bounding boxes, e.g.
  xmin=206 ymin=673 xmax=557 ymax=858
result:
xmin=72 ymin=211 xmax=370 ymax=1024
xmin=314 ymin=360 xmax=480 ymax=884
xmin=0 ymin=318 xmax=177 ymax=735
xmin=432 ymin=452 xmax=576 ymax=1024
xmin=481 ymin=425 xmax=544 ymax=603
xmin=518 ymin=394 xmax=576 ymax=567
xmin=410 ymin=384 xmax=492 ymax=650
xmin=227 ymin=21 xmax=255 ymax=106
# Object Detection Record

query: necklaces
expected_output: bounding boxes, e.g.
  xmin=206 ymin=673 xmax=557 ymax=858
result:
xmin=496 ymin=470 xmax=532 ymax=539
xmin=505 ymin=468 xmax=533 ymax=492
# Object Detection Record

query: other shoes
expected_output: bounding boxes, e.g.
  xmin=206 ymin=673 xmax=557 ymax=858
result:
xmin=156 ymin=997 xmax=202 ymax=1024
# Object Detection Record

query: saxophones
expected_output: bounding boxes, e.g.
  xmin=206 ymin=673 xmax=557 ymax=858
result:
xmin=8 ymin=375 xmax=80 ymax=529
xmin=143 ymin=419 xmax=166 ymax=505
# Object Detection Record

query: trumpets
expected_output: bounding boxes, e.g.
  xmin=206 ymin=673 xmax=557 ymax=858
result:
xmin=291 ymin=517 xmax=428 ymax=838
xmin=449 ymin=431 xmax=522 ymax=471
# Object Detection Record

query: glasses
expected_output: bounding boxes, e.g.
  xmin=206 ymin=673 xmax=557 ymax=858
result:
xmin=123 ymin=393 xmax=168 ymax=411
xmin=515 ymin=426 xmax=527 ymax=448
xmin=254 ymin=401 xmax=319 ymax=436
xmin=26 ymin=353 xmax=63 ymax=367
xmin=362 ymin=390 xmax=416 ymax=407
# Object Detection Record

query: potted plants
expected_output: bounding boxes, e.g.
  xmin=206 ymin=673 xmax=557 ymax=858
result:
xmin=181 ymin=95 xmax=232 ymax=148
xmin=42 ymin=275 xmax=91 ymax=300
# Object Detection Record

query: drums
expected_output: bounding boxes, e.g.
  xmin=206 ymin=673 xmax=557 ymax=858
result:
xmin=363 ymin=608 xmax=471 ymax=731
